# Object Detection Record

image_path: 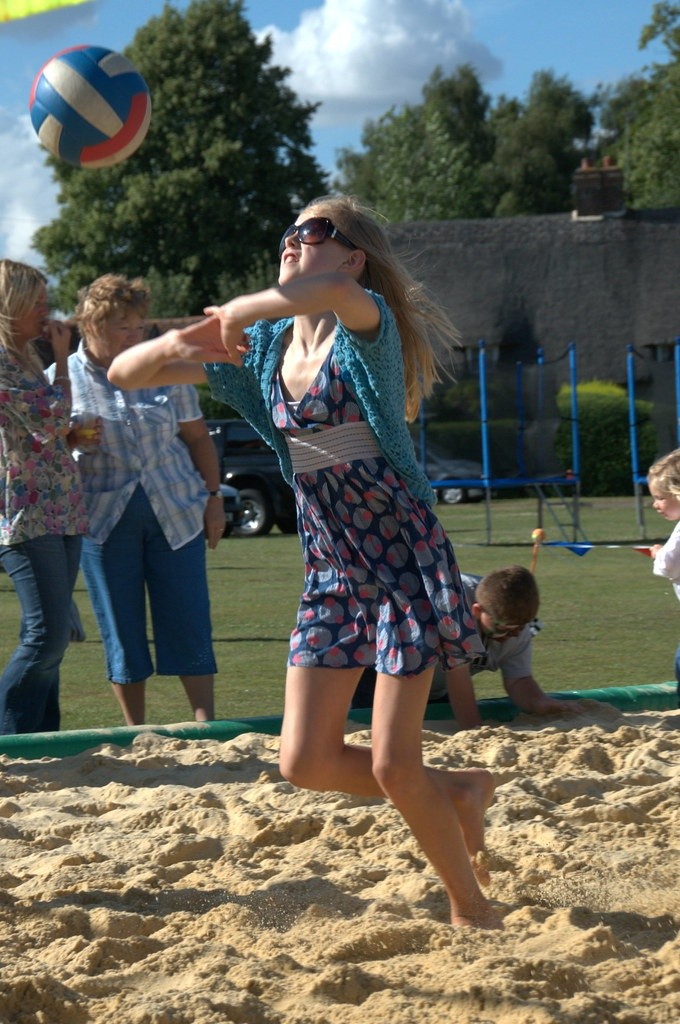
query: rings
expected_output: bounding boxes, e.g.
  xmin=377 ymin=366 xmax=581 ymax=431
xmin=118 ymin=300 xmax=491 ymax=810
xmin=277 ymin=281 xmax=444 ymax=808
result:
xmin=220 ymin=528 xmax=223 ymax=530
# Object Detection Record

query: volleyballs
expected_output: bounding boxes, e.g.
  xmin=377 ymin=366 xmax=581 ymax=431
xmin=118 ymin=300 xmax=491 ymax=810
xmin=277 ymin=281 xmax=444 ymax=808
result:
xmin=30 ymin=43 xmax=154 ymax=170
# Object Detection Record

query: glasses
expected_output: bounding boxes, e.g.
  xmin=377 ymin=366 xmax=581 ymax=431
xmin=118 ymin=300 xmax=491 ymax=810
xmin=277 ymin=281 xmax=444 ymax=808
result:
xmin=278 ymin=217 xmax=369 ymax=267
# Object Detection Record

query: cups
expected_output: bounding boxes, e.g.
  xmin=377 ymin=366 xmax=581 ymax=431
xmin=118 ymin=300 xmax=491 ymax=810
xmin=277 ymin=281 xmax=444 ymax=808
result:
xmin=76 ymin=406 xmax=100 ymax=450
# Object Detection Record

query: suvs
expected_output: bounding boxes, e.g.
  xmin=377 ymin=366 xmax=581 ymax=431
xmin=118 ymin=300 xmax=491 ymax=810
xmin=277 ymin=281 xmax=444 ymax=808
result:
xmin=201 ymin=417 xmax=300 ymax=538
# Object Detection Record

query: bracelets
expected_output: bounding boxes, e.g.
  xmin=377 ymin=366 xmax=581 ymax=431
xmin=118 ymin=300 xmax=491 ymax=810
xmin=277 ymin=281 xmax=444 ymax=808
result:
xmin=54 ymin=376 xmax=71 ymax=382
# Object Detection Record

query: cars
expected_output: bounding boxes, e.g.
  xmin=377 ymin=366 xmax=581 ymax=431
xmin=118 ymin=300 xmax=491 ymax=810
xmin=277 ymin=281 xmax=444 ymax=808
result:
xmin=411 ymin=435 xmax=501 ymax=505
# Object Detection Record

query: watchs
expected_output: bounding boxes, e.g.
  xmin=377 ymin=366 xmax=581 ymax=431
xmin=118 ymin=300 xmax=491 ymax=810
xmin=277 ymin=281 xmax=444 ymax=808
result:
xmin=210 ymin=489 xmax=222 ymax=497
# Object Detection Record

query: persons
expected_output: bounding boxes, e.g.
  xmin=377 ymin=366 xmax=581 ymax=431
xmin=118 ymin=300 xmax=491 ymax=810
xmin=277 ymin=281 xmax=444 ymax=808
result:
xmin=0 ymin=258 xmax=104 ymax=735
xmin=43 ymin=272 xmax=227 ymax=726
xmin=106 ymin=195 xmax=504 ymax=933
xmin=348 ymin=565 xmax=586 ymax=731
xmin=647 ymin=448 xmax=680 ymax=707
